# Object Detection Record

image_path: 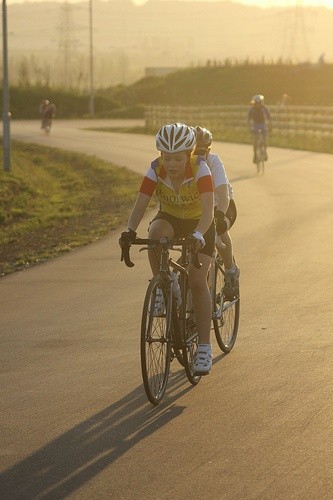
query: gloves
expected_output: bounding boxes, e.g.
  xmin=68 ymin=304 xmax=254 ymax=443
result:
xmin=214 ymin=210 xmax=228 ymax=237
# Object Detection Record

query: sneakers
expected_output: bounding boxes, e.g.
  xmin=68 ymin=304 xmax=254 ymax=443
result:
xmin=148 ymin=286 xmax=165 ymax=316
xmin=224 ymin=264 xmax=240 ymax=301
xmin=193 ymin=344 xmax=212 ymax=374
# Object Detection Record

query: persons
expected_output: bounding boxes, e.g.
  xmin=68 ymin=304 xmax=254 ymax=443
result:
xmin=174 ymin=125 xmax=239 ymax=302
xmin=243 ymin=95 xmax=270 ymax=163
xmin=40 ymin=99 xmax=55 ymax=128
xmin=119 ymin=121 xmax=217 ymax=372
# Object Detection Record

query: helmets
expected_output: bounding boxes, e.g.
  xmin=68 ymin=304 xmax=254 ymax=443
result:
xmin=252 ymin=94 xmax=265 ymax=102
xmin=156 ymin=122 xmax=196 ymax=154
xmin=190 ymin=126 xmax=213 ymax=148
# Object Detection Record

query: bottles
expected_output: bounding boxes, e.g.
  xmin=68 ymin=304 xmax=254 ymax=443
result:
xmin=171 ymin=271 xmax=182 ymax=307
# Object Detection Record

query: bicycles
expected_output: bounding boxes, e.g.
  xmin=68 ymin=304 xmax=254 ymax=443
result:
xmin=114 ymin=230 xmax=241 ymax=407
xmin=252 ymin=131 xmax=269 ymax=175
xmin=40 ymin=112 xmax=53 ymax=135
